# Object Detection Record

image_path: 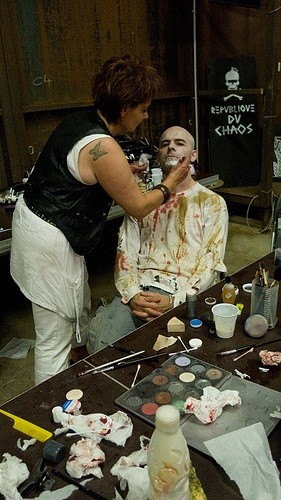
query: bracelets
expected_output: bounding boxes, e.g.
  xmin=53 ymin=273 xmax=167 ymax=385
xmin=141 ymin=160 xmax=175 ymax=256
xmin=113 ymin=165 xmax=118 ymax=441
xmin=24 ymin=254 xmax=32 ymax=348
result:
xmin=153 ymin=183 xmax=170 ymax=207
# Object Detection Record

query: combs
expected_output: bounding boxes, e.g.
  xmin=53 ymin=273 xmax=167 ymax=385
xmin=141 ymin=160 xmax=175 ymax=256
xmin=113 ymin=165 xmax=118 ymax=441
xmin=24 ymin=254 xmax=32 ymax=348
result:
xmin=0 ymin=406 xmax=53 ymax=444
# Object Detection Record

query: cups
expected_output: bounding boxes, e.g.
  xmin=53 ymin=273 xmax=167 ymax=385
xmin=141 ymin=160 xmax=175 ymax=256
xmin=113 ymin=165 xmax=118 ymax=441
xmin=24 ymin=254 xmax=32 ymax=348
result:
xmin=250 ymin=278 xmax=279 ymax=329
xmin=211 ymin=303 xmax=239 ymax=339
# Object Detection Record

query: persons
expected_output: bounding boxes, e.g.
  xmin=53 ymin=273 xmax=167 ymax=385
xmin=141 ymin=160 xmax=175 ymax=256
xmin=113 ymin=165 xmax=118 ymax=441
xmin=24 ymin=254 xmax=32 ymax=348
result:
xmin=10 ymin=56 xmax=191 ymax=390
xmin=85 ymin=126 xmax=229 ymax=357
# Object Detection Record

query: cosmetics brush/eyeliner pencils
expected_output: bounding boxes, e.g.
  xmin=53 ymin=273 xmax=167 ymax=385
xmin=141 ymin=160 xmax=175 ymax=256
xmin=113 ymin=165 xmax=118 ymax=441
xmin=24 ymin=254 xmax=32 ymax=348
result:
xmin=74 ymin=337 xmax=280 ymax=392
xmin=253 ymin=263 xmax=276 ymax=329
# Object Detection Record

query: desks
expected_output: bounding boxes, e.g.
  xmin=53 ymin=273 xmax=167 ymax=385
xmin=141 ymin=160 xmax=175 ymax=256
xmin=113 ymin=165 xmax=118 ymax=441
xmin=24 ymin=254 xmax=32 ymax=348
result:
xmin=0 ymin=249 xmax=281 ymax=500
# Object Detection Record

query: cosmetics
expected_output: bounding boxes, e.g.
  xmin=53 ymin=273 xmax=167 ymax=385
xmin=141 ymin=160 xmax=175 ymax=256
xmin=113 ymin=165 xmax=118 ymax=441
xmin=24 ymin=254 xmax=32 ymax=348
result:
xmin=121 ymin=350 xmax=223 ymax=427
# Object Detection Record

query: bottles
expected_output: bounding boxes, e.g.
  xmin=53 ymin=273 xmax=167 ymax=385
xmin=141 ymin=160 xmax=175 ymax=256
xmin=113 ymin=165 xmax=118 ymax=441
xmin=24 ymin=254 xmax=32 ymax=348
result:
xmin=222 ymin=276 xmax=236 ymax=304
xmin=186 ymin=289 xmax=197 ymax=318
xmin=147 ymin=405 xmax=190 ymax=500
